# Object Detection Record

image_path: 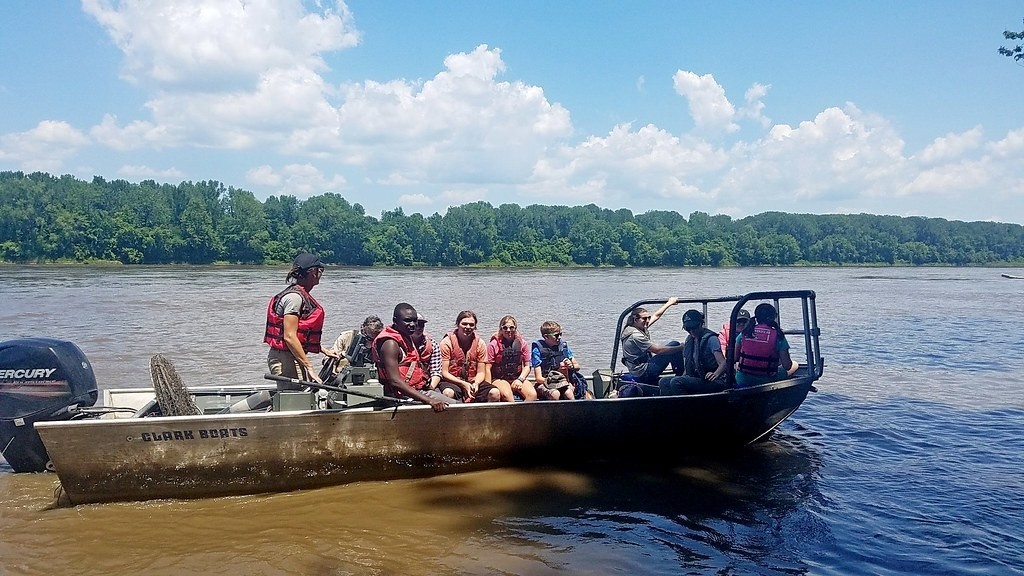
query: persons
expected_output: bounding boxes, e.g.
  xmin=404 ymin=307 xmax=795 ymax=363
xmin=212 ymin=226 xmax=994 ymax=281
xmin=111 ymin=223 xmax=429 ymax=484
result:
xmin=658 ymin=309 xmax=726 ymax=395
xmin=719 ymin=304 xmax=799 ymax=387
xmin=264 ymin=254 xmax=339 ymax=391
xmin=485 ymin=316 xmax=537 ymax=402
xmin=438 ymin=311 xmax=500 ymax=402
xmin=374 ymin=303 xmax=460 ymax=413
xmin=531 ymin=321 xmax=581 ymax=401
xmin=621 ymin=298 xmax=685 ymax=384
xmin=322 ymin=314 xmax=383 ymax=375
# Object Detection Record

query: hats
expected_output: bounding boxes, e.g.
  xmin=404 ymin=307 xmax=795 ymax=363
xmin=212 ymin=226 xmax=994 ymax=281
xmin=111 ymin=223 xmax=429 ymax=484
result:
xmin=293 ymin=253 xmax=326 ymax=270
xmin=544 ymin=370 xmax=569 ymax=389
xmin=737 ymin=310 xmax=750 ymax=319
xmin=682 ymin=310 xmax=704 ymax=330
xmin=417 ymin=313 xmax=427 ymax=323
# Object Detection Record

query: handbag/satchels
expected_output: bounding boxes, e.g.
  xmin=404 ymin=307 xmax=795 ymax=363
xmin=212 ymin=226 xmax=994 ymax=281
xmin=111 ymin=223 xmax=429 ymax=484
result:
xmin=570 ymin=369 xmax=587 ymax=398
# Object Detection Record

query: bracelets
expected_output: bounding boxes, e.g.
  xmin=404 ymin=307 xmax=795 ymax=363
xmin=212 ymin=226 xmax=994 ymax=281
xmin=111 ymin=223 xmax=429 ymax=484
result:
xmin=518 ymin=377 xmax=524 ymax=382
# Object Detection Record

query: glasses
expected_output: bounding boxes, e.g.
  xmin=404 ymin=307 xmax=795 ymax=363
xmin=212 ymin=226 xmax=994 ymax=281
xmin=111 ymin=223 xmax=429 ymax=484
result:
xmin=547 ymin=332 xmax=562 ymax=338
xmin=418 ymin=322 xmax=425 ymax=327
xmin=501 ymin=325 xmax=516 ymax=330
xmin=638 ymin=316 xmax=651 ymax=320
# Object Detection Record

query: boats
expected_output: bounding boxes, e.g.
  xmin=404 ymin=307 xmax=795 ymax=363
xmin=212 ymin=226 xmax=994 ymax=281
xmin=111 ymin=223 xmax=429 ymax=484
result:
xmin=0 ymin=290 xmax=825 ymax=506
